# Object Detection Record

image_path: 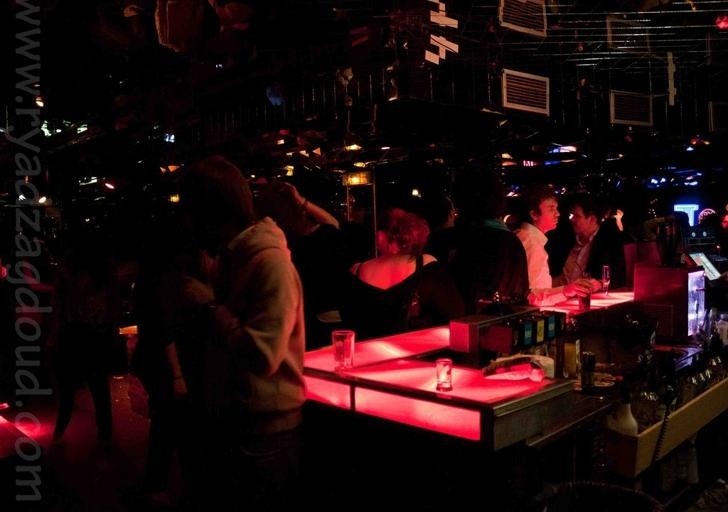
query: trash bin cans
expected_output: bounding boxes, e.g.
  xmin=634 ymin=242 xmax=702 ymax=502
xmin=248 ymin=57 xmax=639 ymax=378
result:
xmin=533 ymin=478 xmax=664 ymax=511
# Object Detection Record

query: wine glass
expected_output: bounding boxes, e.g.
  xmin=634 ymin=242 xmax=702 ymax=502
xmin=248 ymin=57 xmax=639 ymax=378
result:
xmin=601 ymin=264 xmax=611 ymax=298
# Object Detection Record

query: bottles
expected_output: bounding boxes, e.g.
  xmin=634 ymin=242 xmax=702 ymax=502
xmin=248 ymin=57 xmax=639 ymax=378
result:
xmin=616 ymin=395 xmax=638 ymax=434
xmin=563 ymin=322 xmax=582 ymax=376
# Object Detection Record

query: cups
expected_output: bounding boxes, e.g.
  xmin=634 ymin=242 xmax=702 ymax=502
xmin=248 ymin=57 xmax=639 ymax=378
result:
xmin=580 ymin=351 xmax=596 ymax=392
xmin=577 ymin=293 xmax=591 ymax=310
xmin=435 ymin=359 xmax=452 ymax=393
xmin=331 ymin=330 xmax=355 ymax=370
xmin=580 ymin=271 xmax=590 ymax=280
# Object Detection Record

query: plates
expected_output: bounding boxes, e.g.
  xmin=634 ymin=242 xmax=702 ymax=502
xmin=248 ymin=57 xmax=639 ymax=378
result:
xmin=570 ymin=371 xmax=616 ymax=392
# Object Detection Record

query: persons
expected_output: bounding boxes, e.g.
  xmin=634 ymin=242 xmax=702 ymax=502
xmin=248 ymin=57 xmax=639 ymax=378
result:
xmin=52 ymin=132 xmax=728 ymax=512
